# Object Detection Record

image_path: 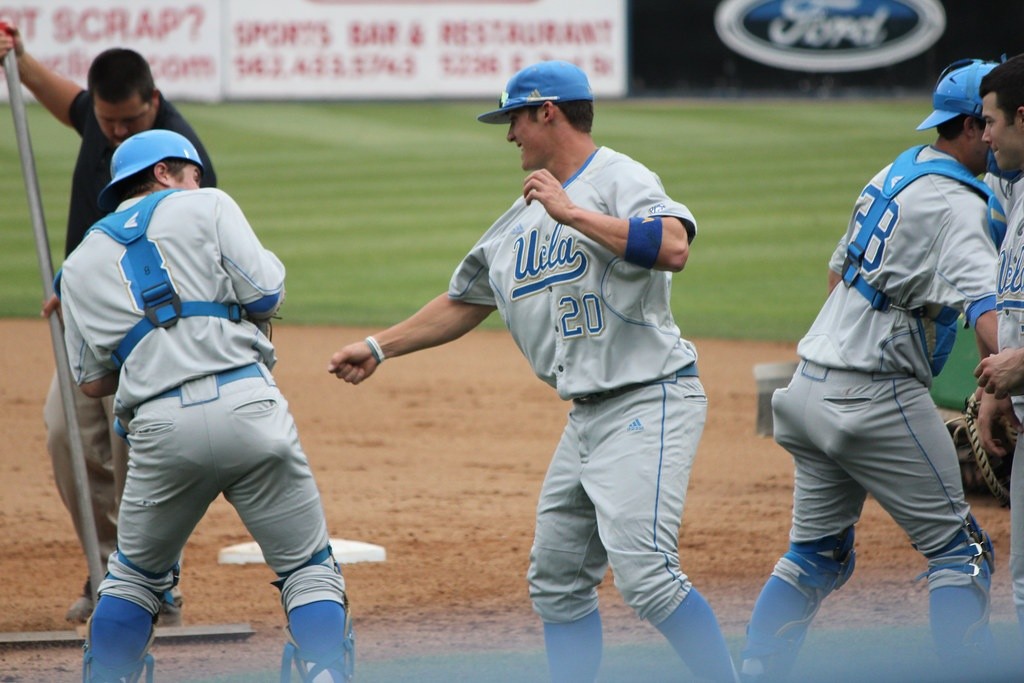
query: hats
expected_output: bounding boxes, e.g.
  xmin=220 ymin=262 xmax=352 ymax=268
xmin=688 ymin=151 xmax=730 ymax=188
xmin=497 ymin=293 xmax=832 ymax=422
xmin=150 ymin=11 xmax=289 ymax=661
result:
xmin=917 ymin=60 xmax=1000 ymax=134
xmin=99 ymin=128 xmax=204 ymax=200
xmin=477 ymin=62 xmax=597 ymax=124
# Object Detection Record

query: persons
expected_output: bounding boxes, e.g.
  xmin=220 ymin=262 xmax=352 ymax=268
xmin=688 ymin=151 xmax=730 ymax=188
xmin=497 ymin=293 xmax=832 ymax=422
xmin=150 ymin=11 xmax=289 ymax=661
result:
xmin=1 ymin=19 xmax=217 ymax=627
xmin=328 ymin=60 xmax=742 ymax=683
xmin=738 ymin=59 xmax=1005 ymax=683
xmin=53 ymin=128 xmax=355 ymax=683
xmin=975 ymin=52 xmax=1024 ymax=627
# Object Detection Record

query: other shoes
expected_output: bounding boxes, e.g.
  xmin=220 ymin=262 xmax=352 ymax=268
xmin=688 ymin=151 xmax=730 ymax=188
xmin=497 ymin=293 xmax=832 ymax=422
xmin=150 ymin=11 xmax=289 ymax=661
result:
xmin=64 ymin=596 xmax=95 ymax=626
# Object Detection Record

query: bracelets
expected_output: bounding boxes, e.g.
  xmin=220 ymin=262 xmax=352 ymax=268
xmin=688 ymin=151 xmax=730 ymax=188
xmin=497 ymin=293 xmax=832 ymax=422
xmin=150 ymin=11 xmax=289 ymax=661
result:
xmin=366 ymin=336 xmax=385 ymax=364
